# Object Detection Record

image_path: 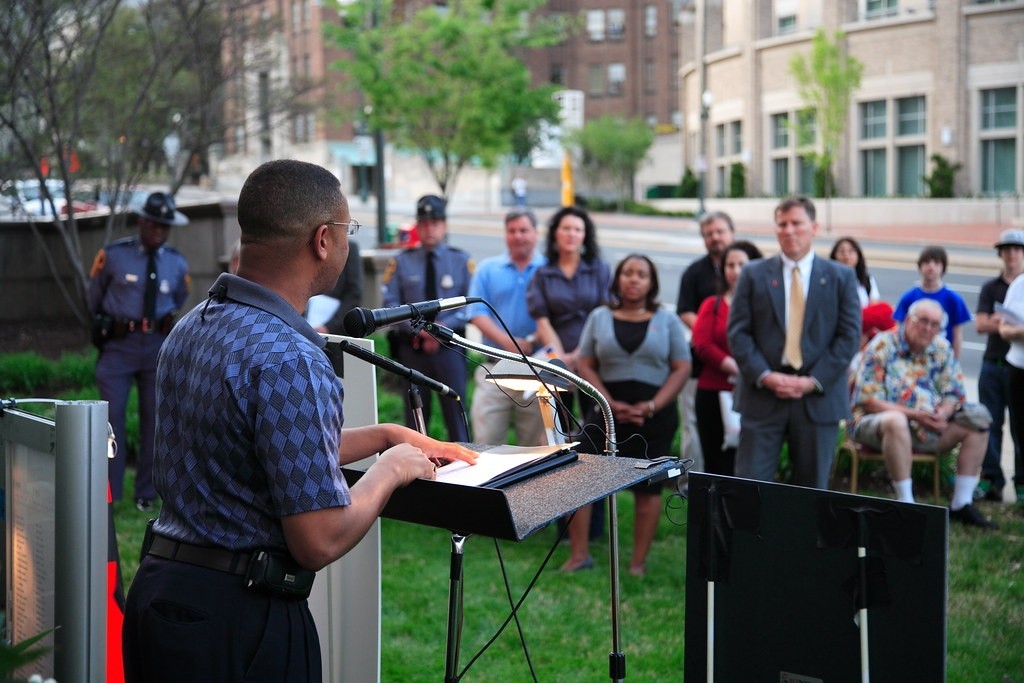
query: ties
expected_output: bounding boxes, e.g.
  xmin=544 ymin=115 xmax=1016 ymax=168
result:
xmin=785 ymin=266 xmax=805 ymax=371
xmin=143 ymin=251 xmax=157 ymax=318
xmin=422 ymin=251 xmax=437 ymax=323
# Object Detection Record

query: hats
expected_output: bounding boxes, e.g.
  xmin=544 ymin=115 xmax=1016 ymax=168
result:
xmin=993 ymin=228 xmax=1024 ymax=247
xmin=416 ymin=195 xmax=448 ymax=218
xmin=134 ymin=192 xmax=189 ymax=226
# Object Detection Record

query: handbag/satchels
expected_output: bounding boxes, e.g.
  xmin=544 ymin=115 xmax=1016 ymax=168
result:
xmin=721 ymin=391 xmax=745 ymax=448
xmin=686 ymin=337 xmax=707 ymax=380
xmin=957 ymin=401 xmax=993 ymax=431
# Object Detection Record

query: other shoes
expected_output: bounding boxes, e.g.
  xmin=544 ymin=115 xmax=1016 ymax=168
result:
xmin=556 ymin=555 xmax=595 ymax=574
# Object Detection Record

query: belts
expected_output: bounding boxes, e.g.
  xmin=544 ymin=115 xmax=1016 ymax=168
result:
xmin=146 ymin=533 xmax=252 ymax=576
xmin=112 ymin=318 xmax=161 ymax=335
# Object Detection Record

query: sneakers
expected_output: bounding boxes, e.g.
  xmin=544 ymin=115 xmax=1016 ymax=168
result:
xmin=946 ymin=502 xmax=999 ymax=533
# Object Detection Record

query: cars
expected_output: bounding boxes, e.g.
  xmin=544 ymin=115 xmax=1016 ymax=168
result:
xmin=0 ymin=178 xmax=151 ymax=218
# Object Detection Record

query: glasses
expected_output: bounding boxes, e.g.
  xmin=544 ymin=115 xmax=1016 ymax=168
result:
xmin=324 ymin=219 xmax=360 ymax=234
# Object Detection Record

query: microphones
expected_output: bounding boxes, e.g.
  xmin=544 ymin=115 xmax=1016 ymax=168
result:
xmin=343 ymin=297 xmax=482 ymax=338
xmin=340 ymin=340 xmax=460 ymax=401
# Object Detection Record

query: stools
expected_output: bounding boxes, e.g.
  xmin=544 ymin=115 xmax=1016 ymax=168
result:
xmin=837 ymin=441 xmax=940 ymax=503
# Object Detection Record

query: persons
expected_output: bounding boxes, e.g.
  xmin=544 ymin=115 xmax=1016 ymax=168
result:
xmin=381 ymin=195 xmax=476 ymax=444
xmin=675 ymin=209 xmax=736 ymax=500
xmin=726 ymin=196 xmax=863 ymax=490
xmin=975 ymin=228 xmax=1024 ymax=502
xmin=511 ymin=171 xmax=528 ymax=205
xmin=122 ymin=159 xmax=481 ymax=683
xmin=691 ymin=240 xmax=764 ymax=477
xmin=88 ymin=192 xmax=191 ymax=513
xmin=301 ymin=241 xmax=365 ymax=336
xmin=846 ymin=298 xmax=1002 ymax=532
xmin=892 ymin=245 xmax=972 ymax=360
xmin=556 ymin=252 xmax=693 ymax=575
xmin=998 ymin=273 xmax=1024 ymax=446
xmin=455 ymin=209 xmax=550 ymax=446
xmin=829 ymin=236 xmax=881 ymax=400
xmin=526 ymin=205 xmax=620 ymax=544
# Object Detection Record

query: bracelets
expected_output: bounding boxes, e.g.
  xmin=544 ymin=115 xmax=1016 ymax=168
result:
xmin=647 ymin=400 xmax=654 ymax=418
xmin=526 ymin=335 xmax=538 ymax=348
